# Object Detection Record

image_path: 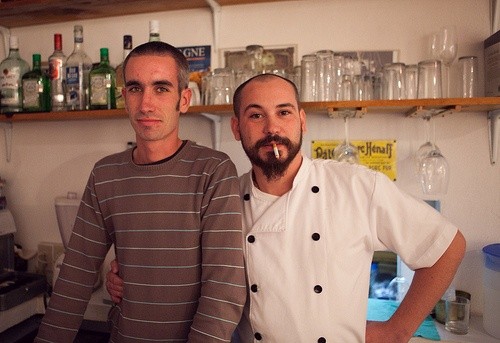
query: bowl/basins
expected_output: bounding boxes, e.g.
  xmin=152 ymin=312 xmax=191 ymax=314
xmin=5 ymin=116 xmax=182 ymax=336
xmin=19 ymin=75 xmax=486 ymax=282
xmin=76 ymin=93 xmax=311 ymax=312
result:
xmin=435 ymin=290 xmax=471 ymax=324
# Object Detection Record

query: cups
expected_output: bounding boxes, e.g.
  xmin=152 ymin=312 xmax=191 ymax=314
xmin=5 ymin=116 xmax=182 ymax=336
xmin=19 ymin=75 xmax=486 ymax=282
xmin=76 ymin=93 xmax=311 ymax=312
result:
xmin=334 ymin=55 xmax=374 ymax=101
xmin=201 ymin=68 xmax=236 ymax=106
xmin=375 ymin=62 xmax=405 ymax=100
xmin=458 ymin=56 xmax=480 ymax=98
xmin=387 ymin=277 xmax=410 ymax=307
xmin=481 ymin=243 xmax=500 ymax=339
xmin=418 ymin=60 xmax=442 ymax=99
xmin=299 ymin=50 xmax=333 ymax=102
xmin=405 ymin=60 xmax=419 ymax=100
xmin=188 ymin=81 xmax=201 ymax=107
xmin=445 ymin=296 xmax=470 ymax=335
xmin=237 ymin=44 xmax=301 ymax=98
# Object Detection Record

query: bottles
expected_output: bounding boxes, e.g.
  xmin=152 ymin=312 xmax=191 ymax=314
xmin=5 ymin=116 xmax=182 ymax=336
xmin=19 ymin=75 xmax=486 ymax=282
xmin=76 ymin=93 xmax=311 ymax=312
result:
xmin=66 ymin=25 xmax=93 ymax=112
xmin=369 ymin=263 xmax=379 ymax=298
xmin=89 ymin=47 xmax=116 ymax=110
xmin=149 ymin=20 xmax=160 ymax=42
xmin=0 ymin=37 xmax=30 ymax=113
xmin=22 ymin=53 xmax=51 ymax=112
xmin=115 ymin=35 xmax=133 ymax=109
xmin=48 ymin=33 xmax=67 ymax=112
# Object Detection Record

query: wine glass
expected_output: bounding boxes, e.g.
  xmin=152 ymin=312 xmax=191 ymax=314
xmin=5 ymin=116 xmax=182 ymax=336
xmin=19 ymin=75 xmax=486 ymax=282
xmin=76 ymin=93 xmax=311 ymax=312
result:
xmin=432 ymin=29 xmax=458 ymax=98
xmin=333 ymin=107 xmax=358 ymax=165
xmin=418 ymin=116 xmax=450 ymax=196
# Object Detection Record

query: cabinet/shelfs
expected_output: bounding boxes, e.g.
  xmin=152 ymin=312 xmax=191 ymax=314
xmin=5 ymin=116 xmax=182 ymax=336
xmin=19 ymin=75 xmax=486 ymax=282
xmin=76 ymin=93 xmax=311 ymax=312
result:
xmin=0 ymin=0 xmax=500 ymax=124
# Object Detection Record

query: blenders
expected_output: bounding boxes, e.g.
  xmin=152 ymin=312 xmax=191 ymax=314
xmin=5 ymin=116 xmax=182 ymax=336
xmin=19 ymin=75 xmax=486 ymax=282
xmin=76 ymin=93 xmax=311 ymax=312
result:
xmin=51 ymin=192 xmax=102 ymax=293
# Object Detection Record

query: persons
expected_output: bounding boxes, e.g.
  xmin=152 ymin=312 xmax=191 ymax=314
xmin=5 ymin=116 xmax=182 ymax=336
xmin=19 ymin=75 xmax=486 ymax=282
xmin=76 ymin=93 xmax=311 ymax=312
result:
xmin=36 ymin=38 xmax=246 ymax=343
xmin=104 ymin=74 xmax=467 ymax=343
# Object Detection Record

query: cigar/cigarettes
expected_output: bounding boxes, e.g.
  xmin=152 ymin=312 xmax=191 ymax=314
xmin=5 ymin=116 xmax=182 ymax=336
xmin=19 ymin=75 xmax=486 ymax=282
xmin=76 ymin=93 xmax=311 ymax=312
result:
xmin=273 ymin=144 xmax=280 ymax=159
